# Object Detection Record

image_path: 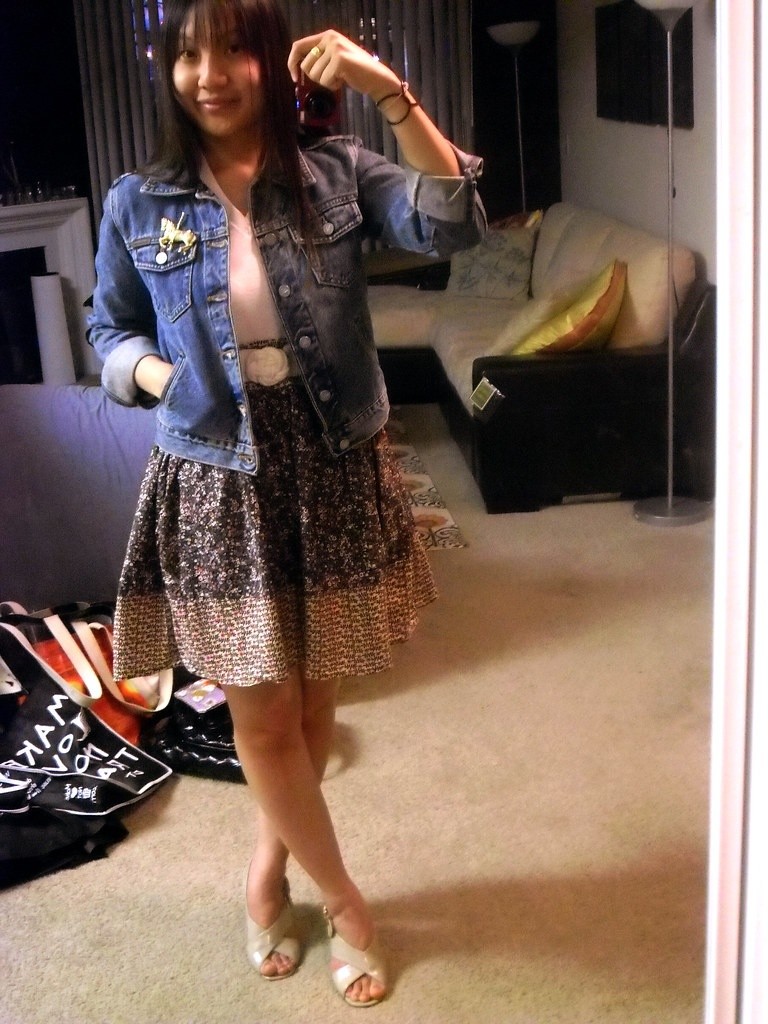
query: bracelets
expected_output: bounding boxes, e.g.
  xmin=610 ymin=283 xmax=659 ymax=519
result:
xmin=376 ymin=81 xmax=421 ymax=126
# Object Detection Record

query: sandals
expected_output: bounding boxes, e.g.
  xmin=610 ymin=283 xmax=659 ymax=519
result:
xmin=322 ymin=896 xmax=387 ymax=1009
xmin=245 ymin=860 xmax=302 ymax=981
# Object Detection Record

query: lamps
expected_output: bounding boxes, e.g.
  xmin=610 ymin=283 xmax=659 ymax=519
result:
xmin=633 ymin=0 xmax=710 ymax=528
xmin=488 ymin=21 xmax=538 ymax=212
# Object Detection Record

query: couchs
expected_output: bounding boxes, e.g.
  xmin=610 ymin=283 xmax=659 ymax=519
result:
xmin=0 ymin=381 xmax=162 ymax=608
xmin=364 ymin=200 xmax=719 ymax=514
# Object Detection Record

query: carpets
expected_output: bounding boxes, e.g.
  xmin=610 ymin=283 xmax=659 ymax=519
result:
xmin=384 ymin=405 xmax=468 ymax=551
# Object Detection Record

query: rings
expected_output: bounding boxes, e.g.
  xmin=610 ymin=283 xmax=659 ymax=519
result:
xmin=311 ymin=47 xmax=322 ymax=57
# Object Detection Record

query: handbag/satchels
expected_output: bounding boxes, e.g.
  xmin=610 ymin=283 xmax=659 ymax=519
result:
xmin=1 ymin=601 xmax=193 ymax=823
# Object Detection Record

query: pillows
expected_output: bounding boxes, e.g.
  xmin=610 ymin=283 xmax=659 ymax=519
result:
xmin=445 ymin=227 xmax=536 ymax=302
xmin=487 ymin=209 xmax=543 ymax=228
xmin=487 ymin=277 xmax=590 ymax=358
xmin=511 ymin=260 xmax=627 ymax=357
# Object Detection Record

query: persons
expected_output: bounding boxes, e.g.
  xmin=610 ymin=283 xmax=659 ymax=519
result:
xmin=86 ymin=0 xmax=488 ymax=1003
